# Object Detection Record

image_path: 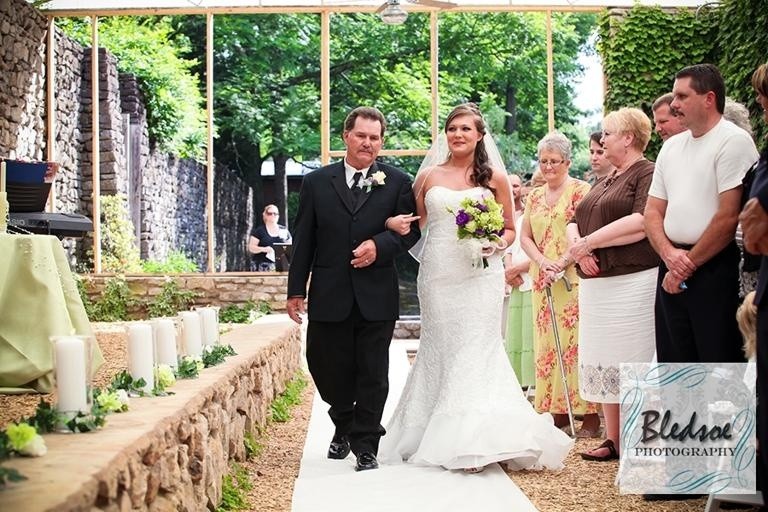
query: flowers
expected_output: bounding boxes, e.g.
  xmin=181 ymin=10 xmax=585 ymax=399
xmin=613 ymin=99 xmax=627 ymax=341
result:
xmin=445 ymin=194 xmax=507 ymax=269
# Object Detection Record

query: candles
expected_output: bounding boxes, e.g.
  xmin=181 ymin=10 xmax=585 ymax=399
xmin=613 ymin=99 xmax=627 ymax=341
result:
xmin=155 ymin=315 xmax=178 ymax=365
xmin=0 ymin=157 xmax=9 ymax=236
xmin=181 ymin=306 xmax=201 ymax=358
xmin=129 ymin=317 xmax=153 ymax=392
xmin=200 ymin=304 xmax=216 ymax=347
xmin=56 ymin=327 xmax=87 ymax=411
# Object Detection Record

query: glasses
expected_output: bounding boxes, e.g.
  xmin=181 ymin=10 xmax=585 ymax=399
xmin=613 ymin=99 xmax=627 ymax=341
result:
xmin=539 ymin=158 xmax=565 ymax=166
xmin=266 ymin=211 xmax=280 ymax=216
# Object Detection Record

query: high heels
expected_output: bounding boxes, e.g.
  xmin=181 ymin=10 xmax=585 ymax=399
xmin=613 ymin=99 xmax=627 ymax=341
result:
xmin=576 ymin=425 xmax=602 ymax=438
xmin=557 ymin=422 xmax=576 ymax=435
xmin=464 ymin=465 xmax=485 ymax=473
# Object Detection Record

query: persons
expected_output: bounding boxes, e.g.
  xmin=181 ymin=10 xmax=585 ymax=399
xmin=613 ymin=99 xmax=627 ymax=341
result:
xmin=505 ymin=180 xmax=535 ymax=387
xmin=517 ymin=131 xmax=603 ymax=438
xmin=286 ymin=107 xmax=422 ymax=471
xmin=384 ymin=104 xmax=576 ymax=473
xmin=652 ymin=93 xmax=689 ymax=145
xmin=500 ymin=174 xmax=525 ymax=351
xmin=566 ymin=107 xmax=660 ymax=461
xmin=249 ymin=205 xmax=291 ymax=272
xmin=736 ymin=293 xmax=757 ymax=398
xmin=641 ymin=65 xmax=762 ymax=500
xmin=586 ymin=132 xmax=618 ymax=186
xmin=739 ymin=65 xmax=767 ymax=512
xmin=723 ymin=96 xmax=762 ymax=299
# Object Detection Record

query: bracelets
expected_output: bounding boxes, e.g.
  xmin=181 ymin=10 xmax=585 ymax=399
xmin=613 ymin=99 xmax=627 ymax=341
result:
xmin=385 ymin=217 xmax=392 ymax=231
xmin=584 ymin=236 xmax=593 ymax=256
xmin=562 ymin=256 xmax=570 ymax=265
xmin=540 ymin=257 xmax=545 ymax=268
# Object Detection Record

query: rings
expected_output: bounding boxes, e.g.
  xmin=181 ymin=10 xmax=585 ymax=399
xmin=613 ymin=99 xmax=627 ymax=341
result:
xmin=365 ymin=259 xmax=369 ymax=264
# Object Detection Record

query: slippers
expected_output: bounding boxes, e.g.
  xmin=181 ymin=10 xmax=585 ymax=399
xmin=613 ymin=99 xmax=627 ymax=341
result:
xmin=578 ymin=439 xmax=620 ymax=462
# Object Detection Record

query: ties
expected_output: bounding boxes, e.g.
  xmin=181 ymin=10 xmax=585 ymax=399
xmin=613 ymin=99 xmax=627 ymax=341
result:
xmin=351 ymin=171 xmax=362 ymax=198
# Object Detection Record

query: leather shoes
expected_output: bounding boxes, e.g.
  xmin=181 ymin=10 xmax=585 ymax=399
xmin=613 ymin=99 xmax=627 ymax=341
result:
xmin=328 ymin=425 xmax=351 ymax=459
xmin=354 ymin=450 xmax=379 ymax=472
